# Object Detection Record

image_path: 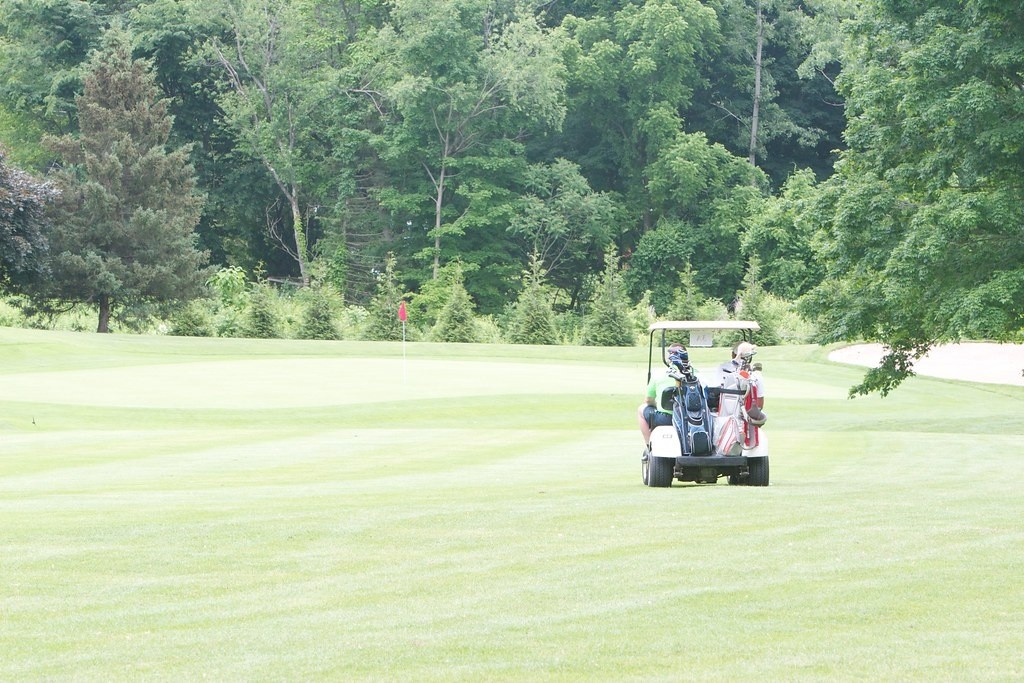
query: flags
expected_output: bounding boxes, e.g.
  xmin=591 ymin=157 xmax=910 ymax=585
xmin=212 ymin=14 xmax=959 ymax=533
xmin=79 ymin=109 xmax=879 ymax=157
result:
xmin=398 ymin=302 xmax=407 ymax=321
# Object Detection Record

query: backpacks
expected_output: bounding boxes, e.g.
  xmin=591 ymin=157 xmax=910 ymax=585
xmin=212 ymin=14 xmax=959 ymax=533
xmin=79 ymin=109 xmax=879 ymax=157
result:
xmin=715 ymin=372 xmax=766 ymax=456
xmin=672 ymin=378 xmax=713 ymax=455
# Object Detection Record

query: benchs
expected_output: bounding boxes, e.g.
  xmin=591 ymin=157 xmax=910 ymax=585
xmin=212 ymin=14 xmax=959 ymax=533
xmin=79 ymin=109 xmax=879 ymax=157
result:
xmin=660 ymin=387 xmax=747 ymax=417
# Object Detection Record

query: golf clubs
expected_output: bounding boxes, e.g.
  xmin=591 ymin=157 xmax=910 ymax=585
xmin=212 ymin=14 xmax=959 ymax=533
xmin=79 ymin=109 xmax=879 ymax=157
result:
xmin=667 ymin=345 xmax=694 ymax=383
xmin=721 ymin=341 xmax=763 ymax=373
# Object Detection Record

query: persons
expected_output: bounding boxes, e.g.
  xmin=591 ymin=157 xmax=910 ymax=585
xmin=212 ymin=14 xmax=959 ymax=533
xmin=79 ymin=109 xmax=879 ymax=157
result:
xmin=714 ymin=342 xmax=764 ymax=409
xmin=639 ymin=344 xmax=704 ymax=464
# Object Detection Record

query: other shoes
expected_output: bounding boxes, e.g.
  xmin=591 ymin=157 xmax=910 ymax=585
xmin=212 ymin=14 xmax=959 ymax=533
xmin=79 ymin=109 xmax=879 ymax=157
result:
xmin=641 ymin=453 xmax=648 ymax=460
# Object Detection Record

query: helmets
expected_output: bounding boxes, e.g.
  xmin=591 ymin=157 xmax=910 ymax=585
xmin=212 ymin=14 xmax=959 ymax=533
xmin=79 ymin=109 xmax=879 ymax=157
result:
xmin=667 ymin=344 xmax=687 ymax=356
xmin=733 ymin=341 xmax=755 ymax=357
xmin=732 ymin=341 xmax=741 ymax=359
